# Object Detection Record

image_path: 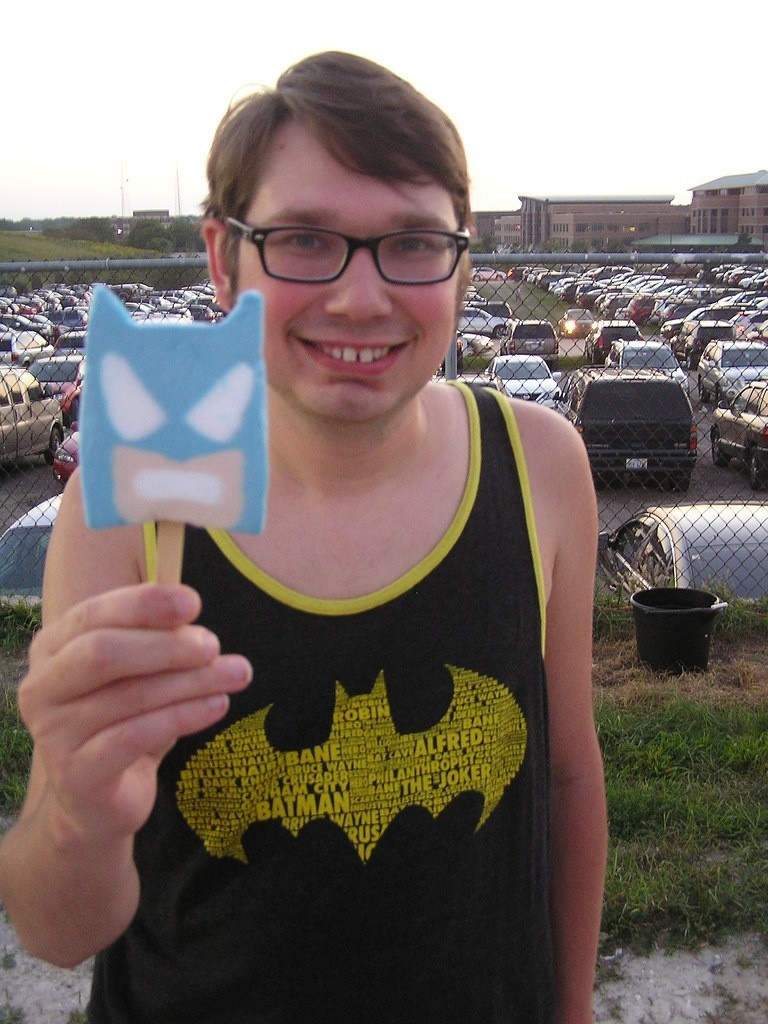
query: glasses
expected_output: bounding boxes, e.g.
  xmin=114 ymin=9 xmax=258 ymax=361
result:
xmin=223 ymin=214 xmax=470 ymax=284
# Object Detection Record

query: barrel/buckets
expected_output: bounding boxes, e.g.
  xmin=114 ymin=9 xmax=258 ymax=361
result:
xmin=628 ymin=585 xmax=728 ymax=675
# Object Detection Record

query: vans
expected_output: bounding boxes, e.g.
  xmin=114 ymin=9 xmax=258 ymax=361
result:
xmin=0 ymin=363 xmax=62 ymax=474
xmin=551 ymin=367 xmax=698 ymax=491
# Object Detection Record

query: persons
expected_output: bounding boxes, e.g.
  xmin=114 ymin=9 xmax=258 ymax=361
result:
xmin=1 ymin=45 xmax=607 ymax=1022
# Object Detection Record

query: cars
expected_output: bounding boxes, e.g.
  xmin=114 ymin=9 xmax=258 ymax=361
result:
xmin=24 ymin=354 xmax=87 ymax=420
xmin=455 ymin=263 xmax=768 ymax=342
xmin=709 ymin=380 xmax=768 ymax=492
xmin=51 ymin=421 xmax=90 ymax=480
xmin=0 ymin=494 xmax=61 ymax=608
xmin=0 ymin=281 xmax=233 ymax=366
xmin=589 ymin=496 xmax=768 ymax=614
xmin=437 ymin=374 xmax=508 ymax=400
xmin=483 ymin=355 xmax=562 ymax=409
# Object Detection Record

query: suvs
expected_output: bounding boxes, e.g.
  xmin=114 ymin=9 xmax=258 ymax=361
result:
xmin=495 ymin=317 xmax=560 ymax=366
xmin=669 ymin=318 xmax=734 ymax=367
xmin=582 ymin=318 xmax=645 ymax=364
xmin=603 ymin=339 xmax=690 ymax=397
xmin=692 ymin=336 xmax=768 ymax=405
